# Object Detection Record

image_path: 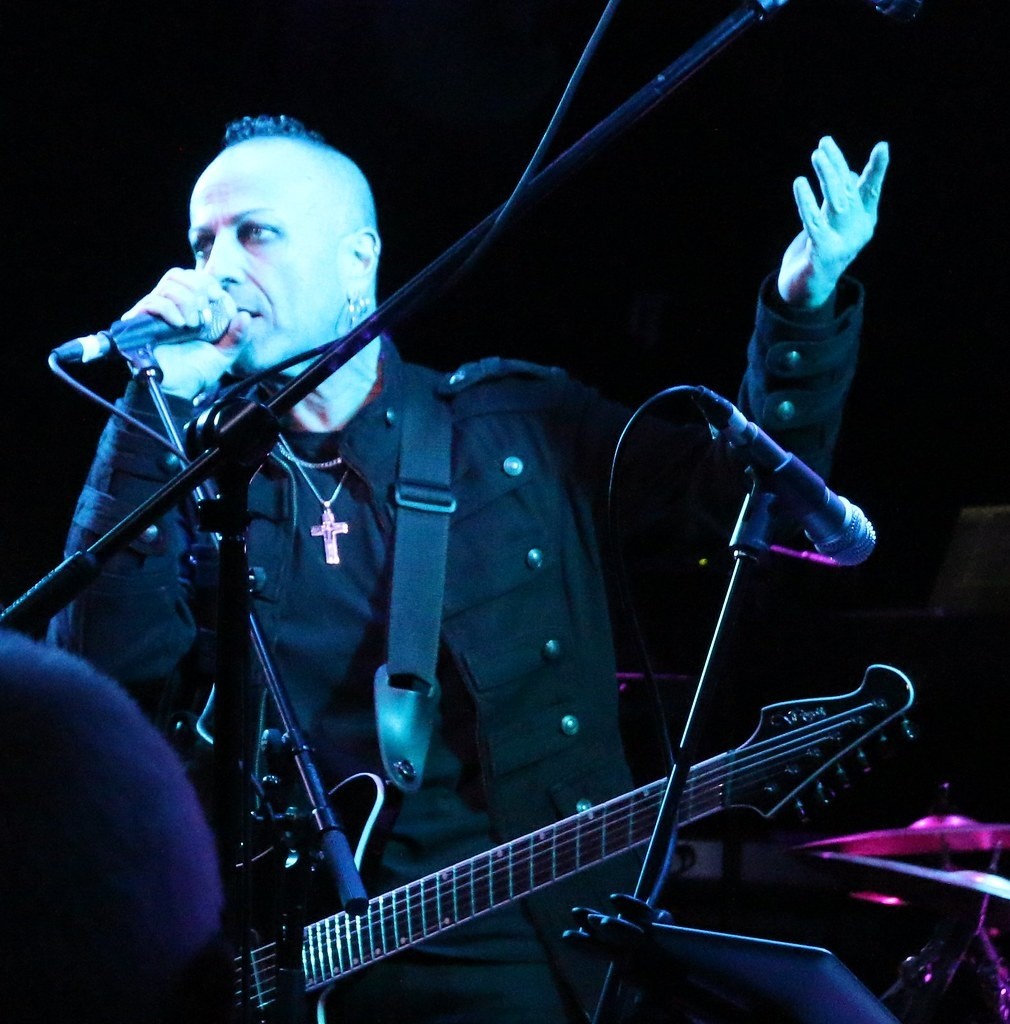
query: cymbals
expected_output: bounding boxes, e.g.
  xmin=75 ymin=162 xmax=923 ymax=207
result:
xmin=814 ymin=849 xmax=1010 ymax=902
xmin=792 ymin=812 xmax=1009 ymax=854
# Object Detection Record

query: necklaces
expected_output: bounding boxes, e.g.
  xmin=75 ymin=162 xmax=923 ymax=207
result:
xmin=276 ymin=435 xmax=353 ymax=566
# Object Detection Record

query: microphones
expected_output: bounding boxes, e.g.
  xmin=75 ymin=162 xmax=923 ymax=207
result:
xmin=692 ymin=386 xmax=877 ymax=566
xmin=54 ymin=290 xmax=237 ymax=362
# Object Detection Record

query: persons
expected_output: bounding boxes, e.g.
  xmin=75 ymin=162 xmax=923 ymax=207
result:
xmin=47 ymin=119 xmax=897 ymax=1024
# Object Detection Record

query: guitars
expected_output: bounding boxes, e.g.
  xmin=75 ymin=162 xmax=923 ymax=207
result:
xmin=213 ymin=658 xmax=912 ymax=1024
